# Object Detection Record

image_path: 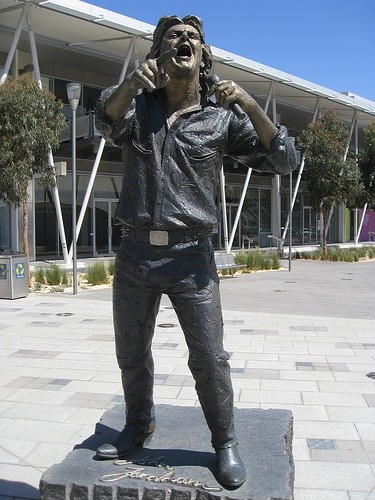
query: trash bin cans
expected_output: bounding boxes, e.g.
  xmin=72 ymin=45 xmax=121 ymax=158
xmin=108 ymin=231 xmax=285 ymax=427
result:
xmin=0 ymin=250 xmax=29 ymax=301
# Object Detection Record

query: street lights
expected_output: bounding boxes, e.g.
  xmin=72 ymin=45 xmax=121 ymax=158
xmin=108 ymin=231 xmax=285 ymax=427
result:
xmin=66 ymin=82 xmax=81 ymax=295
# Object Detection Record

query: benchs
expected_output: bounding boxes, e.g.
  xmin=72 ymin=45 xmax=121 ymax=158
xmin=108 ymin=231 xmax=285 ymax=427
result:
xmin=213 ymin=253 xmax=247 ymax=278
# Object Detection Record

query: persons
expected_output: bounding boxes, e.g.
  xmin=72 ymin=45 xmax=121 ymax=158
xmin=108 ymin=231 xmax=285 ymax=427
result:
xmin=94 ymin=14 xmax=298 ymax=487
